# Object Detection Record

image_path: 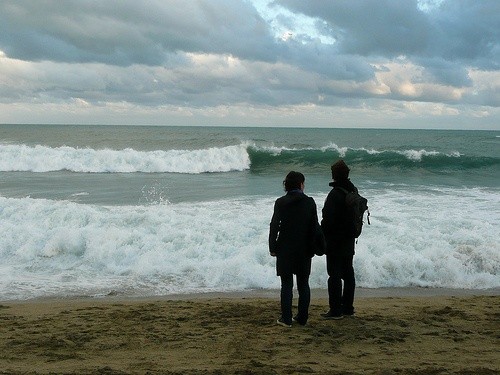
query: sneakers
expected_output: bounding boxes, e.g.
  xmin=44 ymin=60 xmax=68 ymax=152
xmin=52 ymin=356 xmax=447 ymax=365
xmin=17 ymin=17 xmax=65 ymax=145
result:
xmin=320 ymin=309 xmax=343 ymax=319
xmin=343 ymin=312 xmax=353 ymax=317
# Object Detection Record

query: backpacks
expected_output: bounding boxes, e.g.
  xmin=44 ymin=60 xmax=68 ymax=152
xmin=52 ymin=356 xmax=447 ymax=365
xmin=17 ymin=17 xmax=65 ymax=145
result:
xmin=281 ymin=197 xmax=315 ymax=258
xmin=336 ymin=186 xmax=368 ymax=237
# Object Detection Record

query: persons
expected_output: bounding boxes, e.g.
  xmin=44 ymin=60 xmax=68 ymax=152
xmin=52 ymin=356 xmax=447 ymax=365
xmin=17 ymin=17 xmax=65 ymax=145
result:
xmin=269 ymin=171 xmax=326 ymax=328
xmin=319 ymin=160 xmax=359 ymax=320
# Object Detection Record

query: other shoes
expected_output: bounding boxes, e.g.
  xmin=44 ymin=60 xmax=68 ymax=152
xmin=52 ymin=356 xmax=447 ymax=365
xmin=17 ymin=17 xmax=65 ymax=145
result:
xmin=277 ymin=317 xmax=292 ymax=327
xmin=292 ymin=317 xmax=299 ymax=324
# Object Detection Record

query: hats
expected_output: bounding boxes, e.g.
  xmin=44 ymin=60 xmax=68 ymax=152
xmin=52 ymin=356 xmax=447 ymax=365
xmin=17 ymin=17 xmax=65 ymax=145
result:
xmin=331 ymin=160 xmax=346 ymax=176
